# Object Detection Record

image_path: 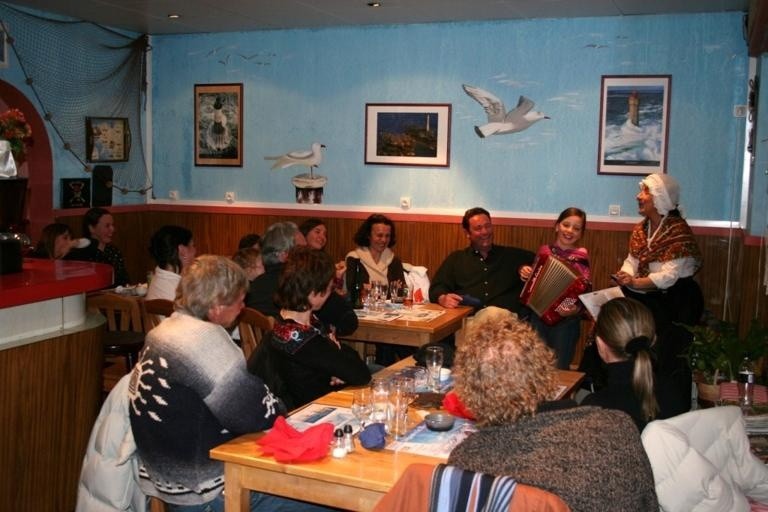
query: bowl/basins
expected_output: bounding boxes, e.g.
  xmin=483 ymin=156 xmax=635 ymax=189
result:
xmin=426 ymin=413 xmax=455 ymax=432
xmin=440 ymin=368 xmax=451 ymax=381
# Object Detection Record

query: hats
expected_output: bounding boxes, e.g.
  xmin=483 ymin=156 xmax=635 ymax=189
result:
xmin=638 ymin=173 xmax=687 ymax=220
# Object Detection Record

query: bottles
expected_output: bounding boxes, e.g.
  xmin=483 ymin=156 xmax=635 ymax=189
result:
xmin=331 ymin=429 xmax=347 ymax=458
xmin=343 ymin=425 xmax=356 ymax=453
xmin=736 ymin=352 xmax=755 ymax=402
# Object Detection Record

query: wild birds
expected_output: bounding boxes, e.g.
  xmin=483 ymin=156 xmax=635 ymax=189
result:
xmin=461 ymin=84 xmax=551 ymax=139
xmin=265 ymin=142 xmax=327 ymax=179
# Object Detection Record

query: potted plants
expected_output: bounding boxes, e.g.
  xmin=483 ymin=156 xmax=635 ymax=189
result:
xmin=672 ymin=315 xmax=764 ymax=402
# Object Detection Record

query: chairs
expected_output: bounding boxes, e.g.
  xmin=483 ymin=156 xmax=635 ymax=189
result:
xmin=138 ymin=298 xmax=176 ymax=334
xmin=238 ymin=308 xmax=274 ymax=362
xmin=86 ymin=293 xmax=142 ymax=331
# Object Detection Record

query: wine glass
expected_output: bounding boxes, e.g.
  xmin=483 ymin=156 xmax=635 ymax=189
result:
xmin=371 ymin=365 xmax=426 ymax=434
xmin=362 ymin=280 xmax=398 ymax=310
xmin=427 ymin=347 xmax=441 ymax=386
xmin=351 ymin=390 xmax=371 ymax=434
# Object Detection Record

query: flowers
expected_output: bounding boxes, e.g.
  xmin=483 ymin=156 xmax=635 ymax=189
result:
xmin=0 ymin=107 xmax=32 ymax=166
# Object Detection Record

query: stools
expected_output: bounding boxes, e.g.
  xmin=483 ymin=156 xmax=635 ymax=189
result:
xmin=100 ymin=330 xmax=145 ymax=376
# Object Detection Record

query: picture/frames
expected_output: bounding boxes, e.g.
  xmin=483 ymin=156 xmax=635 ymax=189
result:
xmin=194 ymin=82 xmax=243 ymax=167
xmin=0 ymin=21 xmax=9 ymax=68
xmin=60 ymin=178 xmax=90 ymax=208
xmin=597 ymin=74 xmax=673 ymax=176
xmin=364 ymin=103 xmax=451 ymax=168
xmin=87 ymin=116 xmax=131 ymax=162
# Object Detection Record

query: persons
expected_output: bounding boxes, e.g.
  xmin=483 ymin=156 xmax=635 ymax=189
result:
xmin=70 ymin=207 xmax=130 ymax=289
xmin=429 ymin=207 xmax=529 ymax=349
xmin=519 ymin=207 xmax=593 ymax=371
xmin=128 ymin=254 xmax=342 ymax=512
xmin=577 ymin=173 xmax=705 ymax=410
xmin=246 ymin=243 xmax=372 ymax=410
xmin=244 ymin=220 xmax=358 ymax=336
xmin=36 ymin=223 xmax=74 ymax=261
xmin=344 ymin=213 xmax=417 ymax=367
xmin=233 ymin=247 xmax=265 ymax=282
xmin=579 ymin=297 xmax=692 ymax=435
xmin=144 ymin=226 xmax=196 ymax=329
xmin=448 ymin=312 xmax=658 ymax=511
xmin=299 ymin=218 xmax=328 ymax=251
